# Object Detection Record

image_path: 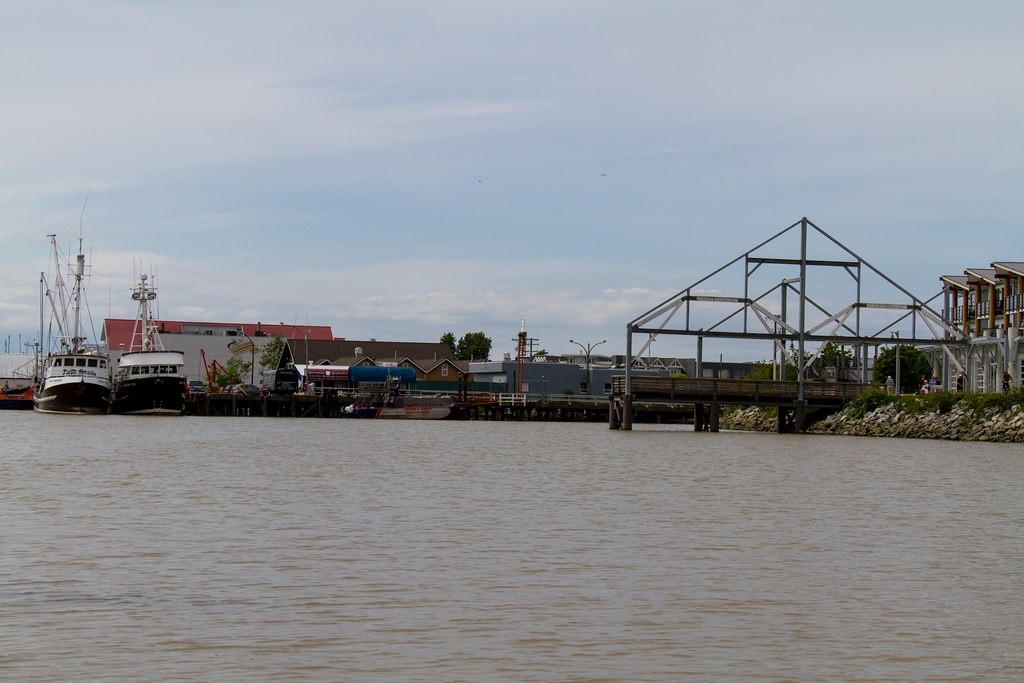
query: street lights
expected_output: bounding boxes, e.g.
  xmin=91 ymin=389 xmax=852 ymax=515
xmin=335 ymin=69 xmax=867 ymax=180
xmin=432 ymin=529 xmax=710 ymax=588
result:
xmin=236 ymin=331 xmax=254 ymax=384
xmin=764 ymin=314 xmax=782 ymax=380
xmin=280 ymin=324 xmax=309 ymax=385
xmin=569 ymin=339 xmax=607 ymax=401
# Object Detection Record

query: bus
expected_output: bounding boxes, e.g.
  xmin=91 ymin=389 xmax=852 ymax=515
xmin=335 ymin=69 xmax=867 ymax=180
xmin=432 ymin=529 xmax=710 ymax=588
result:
xmin=259 ymin=368 xmax=299 ymax=395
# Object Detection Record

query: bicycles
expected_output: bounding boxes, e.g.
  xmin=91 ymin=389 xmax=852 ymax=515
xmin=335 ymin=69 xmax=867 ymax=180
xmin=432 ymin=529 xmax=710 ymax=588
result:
xmin=1002 ymin=381 xmax=1014 ymax=394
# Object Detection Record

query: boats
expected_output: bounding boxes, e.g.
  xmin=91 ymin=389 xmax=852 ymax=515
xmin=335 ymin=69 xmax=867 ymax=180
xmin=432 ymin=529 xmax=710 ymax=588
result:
xmin=0 ymin=189 xmax=189 ymax=416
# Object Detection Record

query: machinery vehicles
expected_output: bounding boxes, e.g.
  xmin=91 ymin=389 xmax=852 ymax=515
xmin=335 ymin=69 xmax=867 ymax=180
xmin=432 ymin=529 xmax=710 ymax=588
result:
xmin=198 ymin=348 xmax=238 ymax=396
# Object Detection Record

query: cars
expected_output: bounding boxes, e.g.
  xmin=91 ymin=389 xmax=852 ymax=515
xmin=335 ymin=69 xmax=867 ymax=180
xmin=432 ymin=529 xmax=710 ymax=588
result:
xmin=226 ymin=384 xmax=261 ymax=396
xmin=187 ymin=380 xmax=206 ymax=392
